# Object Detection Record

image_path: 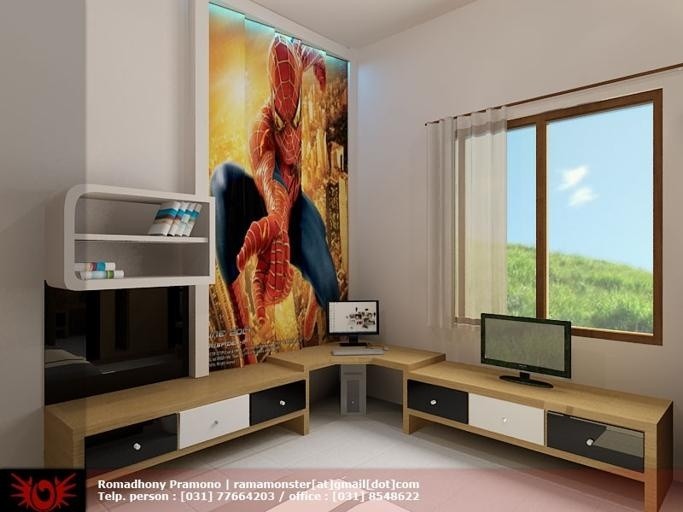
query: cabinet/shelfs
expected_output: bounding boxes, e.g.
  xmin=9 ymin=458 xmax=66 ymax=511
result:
xmin=45 ymin=183 xmax=217 ymax=292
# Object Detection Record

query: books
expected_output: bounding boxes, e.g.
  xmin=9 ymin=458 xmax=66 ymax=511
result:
xmin=74 ymin=261 xmax=125 ymax=280
xmin=149 ymin=201 xmax=202 ymax=236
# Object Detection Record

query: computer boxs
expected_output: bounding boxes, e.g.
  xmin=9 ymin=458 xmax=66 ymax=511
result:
xmin=340 ymin=364 xmax=367 ymax=417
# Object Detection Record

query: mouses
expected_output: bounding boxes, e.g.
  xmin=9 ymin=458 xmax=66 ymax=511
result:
xmin=383 ymin=346 xmax=389 ymax=351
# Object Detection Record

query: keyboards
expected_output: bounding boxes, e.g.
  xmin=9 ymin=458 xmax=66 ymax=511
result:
xmin=331 ymin=347 xmax=385 ymax=356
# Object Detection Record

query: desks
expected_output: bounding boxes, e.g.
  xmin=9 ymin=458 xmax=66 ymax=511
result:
xmin=402 ymin=362 xmax=674 ymax=512
xmin=267 ymin=341 xmax=445 ymax=435
xmin=44 ymin=362 xmax=310 ymax=488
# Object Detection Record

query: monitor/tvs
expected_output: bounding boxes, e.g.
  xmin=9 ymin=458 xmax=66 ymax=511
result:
xmin=44 ymin=279 xmax=190 ymax=405
xmin=326 ymin=299 xmax=380 ymax=347
xmin=480 ymin=313 xmax=572 ymax=389
